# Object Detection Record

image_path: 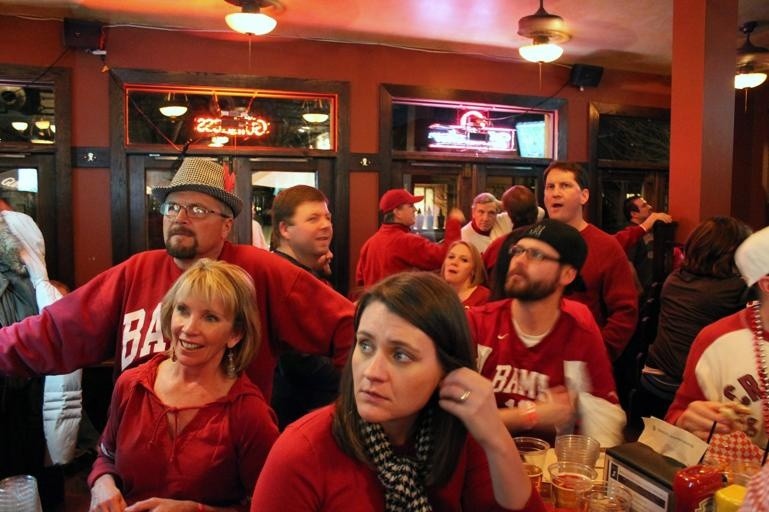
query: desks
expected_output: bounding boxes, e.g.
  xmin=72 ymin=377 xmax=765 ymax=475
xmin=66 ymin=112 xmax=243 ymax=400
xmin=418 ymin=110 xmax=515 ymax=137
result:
xmin=518 ymin=443 xmax=610 ymax=492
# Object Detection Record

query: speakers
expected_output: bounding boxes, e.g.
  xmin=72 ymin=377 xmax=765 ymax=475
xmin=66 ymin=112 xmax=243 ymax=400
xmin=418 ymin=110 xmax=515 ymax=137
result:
xmin=62 ymin=17 xmax=105 ymax=50
xmin=568 ymin=62 xmax=603 ymax=86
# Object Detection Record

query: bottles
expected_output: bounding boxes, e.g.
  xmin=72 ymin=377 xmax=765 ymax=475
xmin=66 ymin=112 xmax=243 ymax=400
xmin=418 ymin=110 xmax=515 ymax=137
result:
xmin=414 ymin=206 xmax=446 ymax=231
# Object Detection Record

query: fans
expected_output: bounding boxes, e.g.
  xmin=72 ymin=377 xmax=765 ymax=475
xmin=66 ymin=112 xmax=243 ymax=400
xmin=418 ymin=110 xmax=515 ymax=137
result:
xmin=220 ymin=1 xmax=361 ymax=35
xmin=724 ymin=20 xmax=769 ymax=70
xmin=516 ymin=1 xmax=638 ymax=51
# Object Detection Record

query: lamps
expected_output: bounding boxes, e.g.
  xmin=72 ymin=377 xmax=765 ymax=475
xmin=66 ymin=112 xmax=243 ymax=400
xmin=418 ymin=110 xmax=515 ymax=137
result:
xmin=732 ymin=68 xmax=769 ymax=114
xmin=514 ymin=35 xmax=568 ymax=92
xmin=222 ymin=5 xmax=282 ymax=65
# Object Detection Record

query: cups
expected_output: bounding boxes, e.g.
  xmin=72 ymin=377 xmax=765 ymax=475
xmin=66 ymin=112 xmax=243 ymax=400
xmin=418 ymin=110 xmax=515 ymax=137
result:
xmin=0 ymin=475 xmax=41 ymax=510
xmin=511 ymin=429 xmax=635 ymax=511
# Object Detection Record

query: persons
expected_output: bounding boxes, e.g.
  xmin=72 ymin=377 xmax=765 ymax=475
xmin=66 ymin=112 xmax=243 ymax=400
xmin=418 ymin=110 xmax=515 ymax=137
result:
xmin=1 ymin=155 xmax=769 ymax=512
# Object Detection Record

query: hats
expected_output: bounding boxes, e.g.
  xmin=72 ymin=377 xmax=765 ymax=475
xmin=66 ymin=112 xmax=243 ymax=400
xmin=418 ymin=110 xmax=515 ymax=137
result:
xmin=516 ymin=219 xmax=587 ymax=291
xmin=734 ymin=226 xmax=769 ymax=302
xmin=152 ymin=157 xmax=243 ymax=217
xmin=380 ymin=189 xmax=424 ymax=214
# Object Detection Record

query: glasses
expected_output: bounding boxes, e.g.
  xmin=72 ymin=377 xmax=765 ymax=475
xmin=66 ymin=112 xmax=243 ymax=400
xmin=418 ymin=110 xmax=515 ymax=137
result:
xmin=160 ymin=203 xmax=229 ymax=218
xmin=509 ymin=245 xmax=560 ymax=262
